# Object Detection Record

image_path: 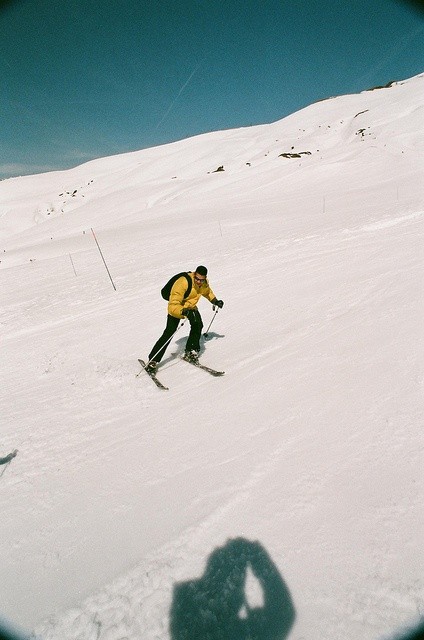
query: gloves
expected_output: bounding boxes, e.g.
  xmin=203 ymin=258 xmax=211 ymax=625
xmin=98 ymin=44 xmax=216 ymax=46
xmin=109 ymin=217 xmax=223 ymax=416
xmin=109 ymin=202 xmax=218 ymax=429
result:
xmin=211 ymin=297 xmax=223 ymax=308
xmin=182 ymin=309 xmax=189 ymax=315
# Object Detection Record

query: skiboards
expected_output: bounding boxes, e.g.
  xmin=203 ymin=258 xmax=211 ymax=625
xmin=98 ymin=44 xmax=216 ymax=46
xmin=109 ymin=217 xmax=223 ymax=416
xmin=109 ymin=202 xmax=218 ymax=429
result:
xmin=138 ymin=353 xmax=225 ymax=390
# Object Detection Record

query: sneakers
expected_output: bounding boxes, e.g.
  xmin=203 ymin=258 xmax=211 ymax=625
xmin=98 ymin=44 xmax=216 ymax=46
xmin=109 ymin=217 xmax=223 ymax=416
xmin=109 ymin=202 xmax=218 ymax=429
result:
xmin=148 ymin=361 xmax=156 ymax=372
xmin=185 ymin=350 xmax=198 ymax=362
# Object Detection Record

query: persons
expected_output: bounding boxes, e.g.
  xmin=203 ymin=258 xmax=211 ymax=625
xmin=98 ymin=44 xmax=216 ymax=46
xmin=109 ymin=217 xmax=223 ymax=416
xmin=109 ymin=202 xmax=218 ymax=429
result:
xmin=146 ymin=266 xmax=223 ymax=375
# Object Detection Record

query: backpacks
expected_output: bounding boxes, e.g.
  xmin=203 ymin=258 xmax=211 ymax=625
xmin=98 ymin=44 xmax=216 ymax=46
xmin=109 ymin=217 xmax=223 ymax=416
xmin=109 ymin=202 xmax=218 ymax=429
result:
xmin=161 ymin=271 xmax=192 ymax=301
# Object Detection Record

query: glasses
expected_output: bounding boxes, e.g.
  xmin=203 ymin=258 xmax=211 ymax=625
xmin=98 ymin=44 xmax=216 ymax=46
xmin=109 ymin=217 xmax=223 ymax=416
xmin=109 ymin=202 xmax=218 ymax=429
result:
xmin=193 ymin=274 xmax=206 ymax=283
